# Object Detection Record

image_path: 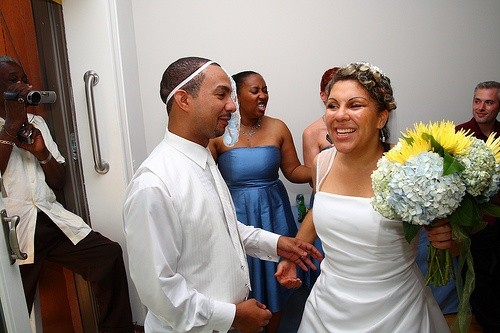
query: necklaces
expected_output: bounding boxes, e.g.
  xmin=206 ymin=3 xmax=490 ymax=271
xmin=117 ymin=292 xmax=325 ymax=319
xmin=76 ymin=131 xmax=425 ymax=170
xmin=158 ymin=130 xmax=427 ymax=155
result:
xmin=237 ymin=116 xmax=263 ymax=139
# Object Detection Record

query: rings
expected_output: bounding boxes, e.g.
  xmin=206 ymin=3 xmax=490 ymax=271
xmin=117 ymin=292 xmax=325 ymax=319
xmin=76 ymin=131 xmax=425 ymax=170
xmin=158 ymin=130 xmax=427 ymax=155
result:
xmin=299 ymin=255 xmax=305 ymax=260
xmin=17 ymin=97 xmax=25 ymax=104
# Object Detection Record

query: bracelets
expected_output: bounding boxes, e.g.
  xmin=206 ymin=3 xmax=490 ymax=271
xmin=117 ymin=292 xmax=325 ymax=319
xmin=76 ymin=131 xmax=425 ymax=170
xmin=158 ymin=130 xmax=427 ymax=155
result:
xmin=1 ymin=124 xmax=18 ymax=139
xmin=0 ymin=140 xmax=16 ymax=147
xmin=37 ymin=151 xmax=52 ymax=165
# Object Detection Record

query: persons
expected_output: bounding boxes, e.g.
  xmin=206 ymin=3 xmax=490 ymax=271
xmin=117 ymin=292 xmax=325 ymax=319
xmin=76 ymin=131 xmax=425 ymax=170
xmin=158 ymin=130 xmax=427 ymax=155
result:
xmin=455 ymin=80 xmax=500 ymax=333
xmin=207 ymin=70 xmax=314 ymax=333
xmin=302 ymin=66 xmax=343 ymax=290
xmin=273 ymin=61 xmax=463 ymax=333
xmin=122 ymin=57 xmax=323 ymax=333
xmin=0 ymin=56 xmax=135 ymax=333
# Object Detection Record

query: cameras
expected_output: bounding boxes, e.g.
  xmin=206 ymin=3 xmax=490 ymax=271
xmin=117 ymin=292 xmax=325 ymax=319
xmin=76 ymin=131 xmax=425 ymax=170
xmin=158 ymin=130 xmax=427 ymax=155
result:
xmin=3 ymin=88 xmax=57 ymax=106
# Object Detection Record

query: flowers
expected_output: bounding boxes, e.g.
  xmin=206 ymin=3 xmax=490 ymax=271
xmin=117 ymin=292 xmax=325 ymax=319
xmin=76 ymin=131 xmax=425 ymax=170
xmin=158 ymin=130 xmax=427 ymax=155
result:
xmin=370 ymin=119 xmax=500 ymax=288
